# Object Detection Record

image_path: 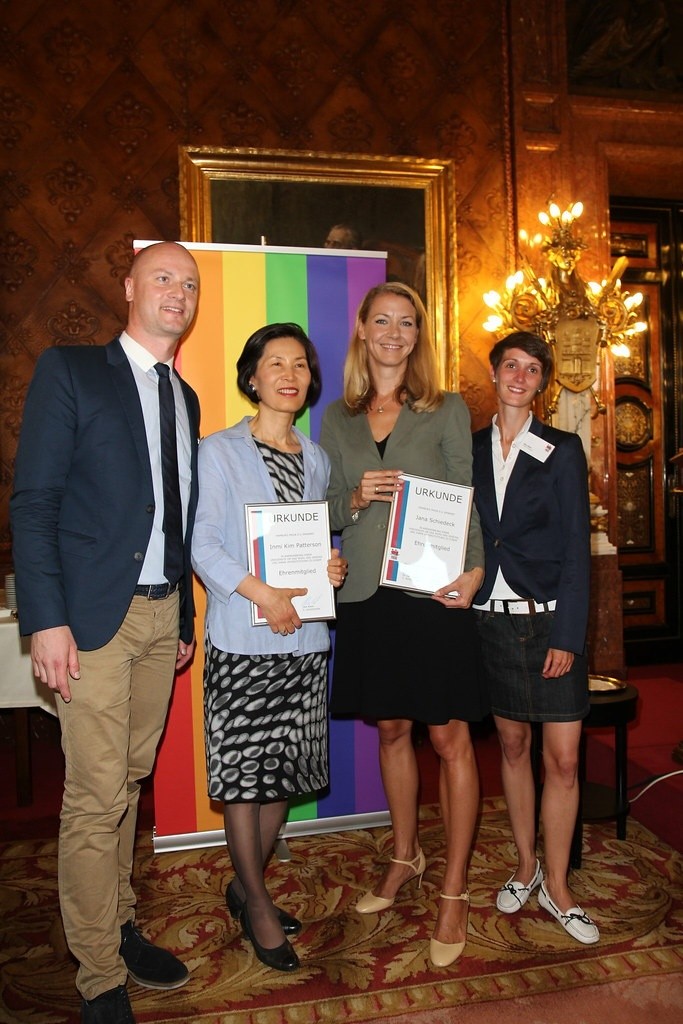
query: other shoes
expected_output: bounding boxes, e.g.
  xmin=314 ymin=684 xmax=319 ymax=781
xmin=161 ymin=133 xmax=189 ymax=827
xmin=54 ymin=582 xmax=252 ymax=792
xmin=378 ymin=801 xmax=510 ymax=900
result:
xmin=117 ymin=919 xmax=191 ymax=991
xmin=496 ymin=857 xmax=543 ymax=913
xmin=83 ymin=984 xmax=136 ymax=1024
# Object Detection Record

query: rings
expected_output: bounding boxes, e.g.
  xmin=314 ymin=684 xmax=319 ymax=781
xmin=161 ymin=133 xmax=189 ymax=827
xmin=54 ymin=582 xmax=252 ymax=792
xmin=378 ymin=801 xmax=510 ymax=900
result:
xmin=341 ymin=575 xmax=345 ymax=581
xmin=375 ymin=485 xmax=379 ymax=493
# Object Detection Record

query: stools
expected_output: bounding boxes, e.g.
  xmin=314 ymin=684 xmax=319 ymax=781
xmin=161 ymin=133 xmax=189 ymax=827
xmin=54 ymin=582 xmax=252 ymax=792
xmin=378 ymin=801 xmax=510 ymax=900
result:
xmin=529 ymin=675 xmax=640 ymax=868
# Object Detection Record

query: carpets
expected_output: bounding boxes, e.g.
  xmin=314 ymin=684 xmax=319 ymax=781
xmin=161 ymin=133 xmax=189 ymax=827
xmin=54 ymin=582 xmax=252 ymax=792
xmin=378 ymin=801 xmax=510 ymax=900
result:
xmin=0 ymin=795 xmax=683 ymax=1024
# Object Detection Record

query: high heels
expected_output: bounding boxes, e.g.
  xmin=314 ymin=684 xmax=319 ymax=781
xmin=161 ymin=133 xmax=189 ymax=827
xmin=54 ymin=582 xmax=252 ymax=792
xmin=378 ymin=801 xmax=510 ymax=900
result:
xmin=430 ymin=891 xmax=470 ymax=968
xmin=356 ymin=847 xmax=426 ymax=913
xmin=239 ymin=899 xmax=301 ymax=972
xmin=226 ymin=882 xmax=302 ymax=936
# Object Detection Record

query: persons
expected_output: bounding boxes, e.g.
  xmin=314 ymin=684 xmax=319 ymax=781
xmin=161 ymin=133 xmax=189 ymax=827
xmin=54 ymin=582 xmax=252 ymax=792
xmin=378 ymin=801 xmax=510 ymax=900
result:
xmin=317 ymin=281 xmax=485 ymax=967
xmin=10 ymin=242 xmax=201 ymax=1024
xmin=470 ymin=331 xmax=600 ymax=944
xmin=190 ymin=322 xmax=349 ymax=972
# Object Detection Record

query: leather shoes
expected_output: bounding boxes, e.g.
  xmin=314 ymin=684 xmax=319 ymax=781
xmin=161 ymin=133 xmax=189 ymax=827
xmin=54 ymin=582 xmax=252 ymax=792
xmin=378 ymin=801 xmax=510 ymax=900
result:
xmin=538 ymin=880 xmax=601 ymax=944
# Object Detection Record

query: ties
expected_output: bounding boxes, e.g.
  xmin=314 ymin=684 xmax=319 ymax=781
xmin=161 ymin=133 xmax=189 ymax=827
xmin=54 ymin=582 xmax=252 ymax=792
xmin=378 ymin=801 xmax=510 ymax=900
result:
xmin=153 ymin=360 xmax=185 ymax=587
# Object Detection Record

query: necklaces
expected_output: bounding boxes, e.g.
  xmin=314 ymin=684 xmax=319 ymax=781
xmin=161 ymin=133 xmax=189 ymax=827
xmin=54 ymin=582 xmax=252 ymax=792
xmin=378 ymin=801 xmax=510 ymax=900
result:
xmin=376 ymin=398 xmax=391 ymax=413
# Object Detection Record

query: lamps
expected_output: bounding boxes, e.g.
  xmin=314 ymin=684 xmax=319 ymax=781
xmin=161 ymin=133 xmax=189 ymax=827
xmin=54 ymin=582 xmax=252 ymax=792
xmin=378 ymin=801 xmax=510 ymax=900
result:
xmin=482 ymin=202 xmax=647 ymax=419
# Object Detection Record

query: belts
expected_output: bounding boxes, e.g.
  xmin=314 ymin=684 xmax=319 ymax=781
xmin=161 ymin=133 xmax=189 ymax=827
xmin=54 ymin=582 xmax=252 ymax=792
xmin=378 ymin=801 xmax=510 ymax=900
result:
xmin=134 ymin=581 xmax=178 ymax=600
xmin=472 ymin=598 xmax=557 ymax=615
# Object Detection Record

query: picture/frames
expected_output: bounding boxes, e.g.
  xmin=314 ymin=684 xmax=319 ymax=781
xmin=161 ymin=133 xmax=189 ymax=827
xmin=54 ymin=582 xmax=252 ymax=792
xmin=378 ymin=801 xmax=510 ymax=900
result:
xmin=176 ymin=145 xmax=459 ymax=395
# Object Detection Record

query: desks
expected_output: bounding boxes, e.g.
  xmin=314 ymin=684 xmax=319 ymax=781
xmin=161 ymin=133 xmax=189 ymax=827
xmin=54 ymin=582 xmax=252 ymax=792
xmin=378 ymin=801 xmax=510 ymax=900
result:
xmin=0 ymin=588 xmax=58 ymax=808
